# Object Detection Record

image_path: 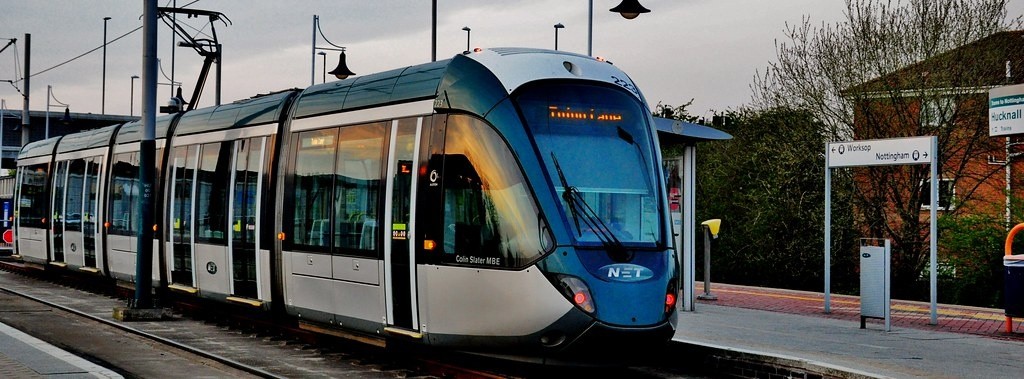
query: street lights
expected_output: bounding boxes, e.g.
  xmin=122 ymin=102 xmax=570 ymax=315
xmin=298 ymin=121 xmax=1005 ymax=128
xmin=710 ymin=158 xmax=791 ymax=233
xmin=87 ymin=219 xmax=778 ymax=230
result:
xmin=553 ymin=23 xmax=565 ymax=50
xmin=310 ymin=14 xmax=356 ymax=86
xmin=317 ymin=52 xmax=326 ymax=83
xmin=462 ymin=26 xmax=471 ymax=51
xmin=101 ymin=16 xmax=112 ymax=112
xmin=130 ymin=75 xmax=140 ymax=116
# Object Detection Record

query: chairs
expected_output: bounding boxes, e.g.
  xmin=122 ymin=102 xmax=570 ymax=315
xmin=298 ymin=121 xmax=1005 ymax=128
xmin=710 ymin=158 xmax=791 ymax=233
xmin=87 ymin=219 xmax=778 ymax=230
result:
xmin=310 ymin=212 xmax=379 ymax=249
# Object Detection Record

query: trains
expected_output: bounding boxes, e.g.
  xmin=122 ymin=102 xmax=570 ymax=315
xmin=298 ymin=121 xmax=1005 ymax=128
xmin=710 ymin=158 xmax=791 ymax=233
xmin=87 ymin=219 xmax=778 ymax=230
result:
xmin=0 ymin=46 xmax=682 ymax=379
